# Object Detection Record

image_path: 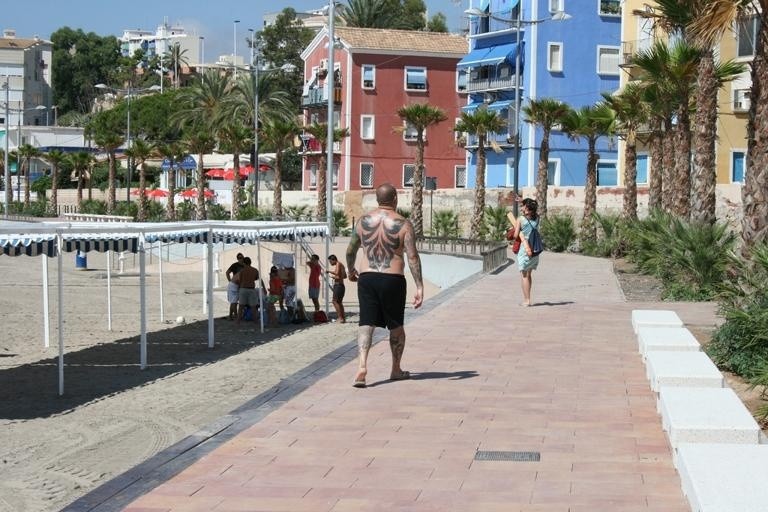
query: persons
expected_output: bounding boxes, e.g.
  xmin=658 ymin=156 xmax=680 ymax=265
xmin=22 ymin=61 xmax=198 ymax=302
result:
xmin=344 ymin=183 xmax=423 ymax=386
xmin=305 ymin=254 xmax=321 ymax=311
xmin=225 ymin=253 xmax=243 ymax=317
xmin=276 ymin=266 xmax=285 ymax=311
xmin=514 ymin=198 xmax=541 ymax=308
xmin=325 ymin=254 xmax=347 ymax=323
xmin=235 ymin=256 xmax=258 ymax=324
xmin=268 ymin=266 xmax=284 ymax=324
xmin=283 ymin=266 xmax=296 ymax=321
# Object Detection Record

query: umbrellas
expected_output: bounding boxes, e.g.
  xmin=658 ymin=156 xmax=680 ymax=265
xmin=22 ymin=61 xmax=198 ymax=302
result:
xmin=236 ymin=166 xmax=255 ymax=185
xmin=246 ymin=163 xmax=273 ymax=190
xmin=176 ymin=187 xmax=216 ymax=204
xmin=143 ymin=189 xmax=169 ymax=201
xmin=130 ymin=188 xmax=150 ymax=198
xmin=202 ymin=169 xmax=224 ymax=181
xmin=223 ymin=169 xmax=243 ymax=186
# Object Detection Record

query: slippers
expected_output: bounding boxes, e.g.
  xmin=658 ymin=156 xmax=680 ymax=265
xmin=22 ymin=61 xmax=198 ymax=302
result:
xmin=390 ymin=370 xmax=410 ymax=380
xmin=353 ymin=378 xmax=368 ymax=388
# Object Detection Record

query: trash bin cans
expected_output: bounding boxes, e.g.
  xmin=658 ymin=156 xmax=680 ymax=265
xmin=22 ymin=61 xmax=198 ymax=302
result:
xmin=75 ymin=250 xmax=87 ymax=270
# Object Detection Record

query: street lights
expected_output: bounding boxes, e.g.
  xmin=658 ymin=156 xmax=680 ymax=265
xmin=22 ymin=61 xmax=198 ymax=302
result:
xmin=463 ymin=8 xmax=572 ymax=224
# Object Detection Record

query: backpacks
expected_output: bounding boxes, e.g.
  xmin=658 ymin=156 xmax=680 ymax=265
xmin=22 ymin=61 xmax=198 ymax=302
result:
xmin=523 ymin=213 xmax=544 ymax=255
